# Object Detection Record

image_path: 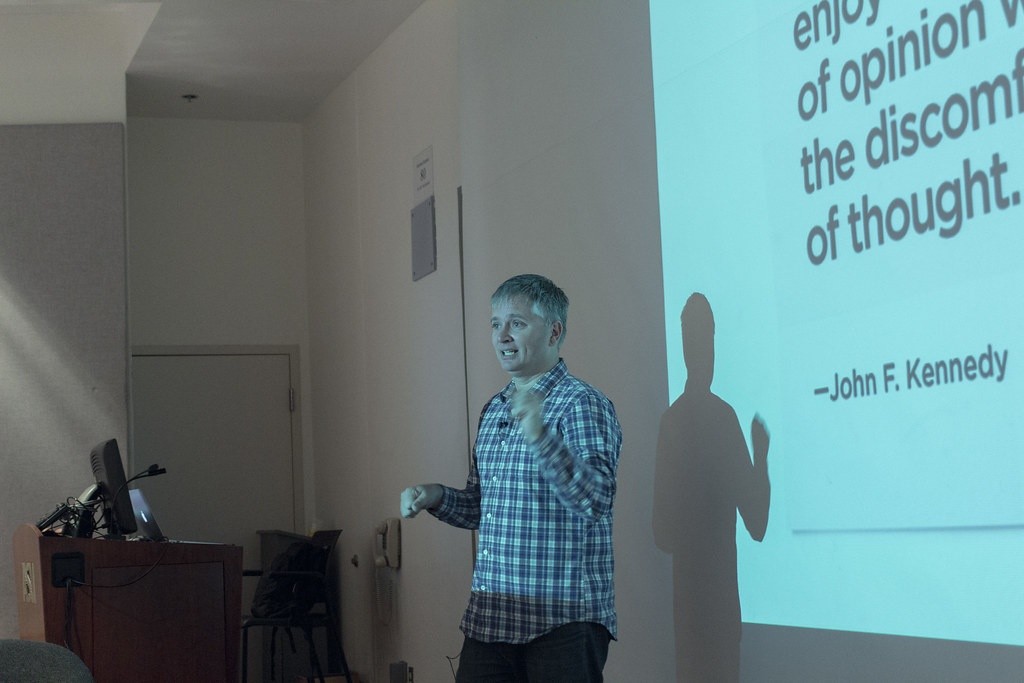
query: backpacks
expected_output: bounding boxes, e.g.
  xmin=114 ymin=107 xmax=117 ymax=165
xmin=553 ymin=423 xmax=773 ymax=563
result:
xmin=251 ymin=542 xmax=327 ymax=623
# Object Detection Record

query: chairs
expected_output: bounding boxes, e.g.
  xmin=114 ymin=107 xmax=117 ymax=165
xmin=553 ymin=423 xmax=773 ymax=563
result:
xmin=0 ymin=639 xmax=93 ymax=683
xmin=239 ymin=530 xmax=352 ymax=683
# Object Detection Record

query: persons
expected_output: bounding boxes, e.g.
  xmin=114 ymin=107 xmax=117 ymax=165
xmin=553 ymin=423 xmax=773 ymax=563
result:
xmin=398 ymin=274 xmax=621 ymax=683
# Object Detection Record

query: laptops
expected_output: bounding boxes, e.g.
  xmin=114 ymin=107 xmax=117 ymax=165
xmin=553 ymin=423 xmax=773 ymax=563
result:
xmin=128 ymin=488 xmax=224 ymax=546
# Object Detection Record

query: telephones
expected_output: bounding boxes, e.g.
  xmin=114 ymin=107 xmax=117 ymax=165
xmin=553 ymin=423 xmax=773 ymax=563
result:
xmin=371 ymin=518 xmax=400 ymax=568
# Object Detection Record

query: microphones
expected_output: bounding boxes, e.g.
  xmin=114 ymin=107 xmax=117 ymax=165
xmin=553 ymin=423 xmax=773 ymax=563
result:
xmin=105 ymin=463 xmax=167 ymax=540
xmin=499 ymin=421 xmax=509 ymax=428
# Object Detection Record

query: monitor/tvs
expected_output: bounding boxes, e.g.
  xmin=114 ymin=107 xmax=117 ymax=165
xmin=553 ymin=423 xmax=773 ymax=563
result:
xmin=62 ymin=439 xmax=137 ymax=539
